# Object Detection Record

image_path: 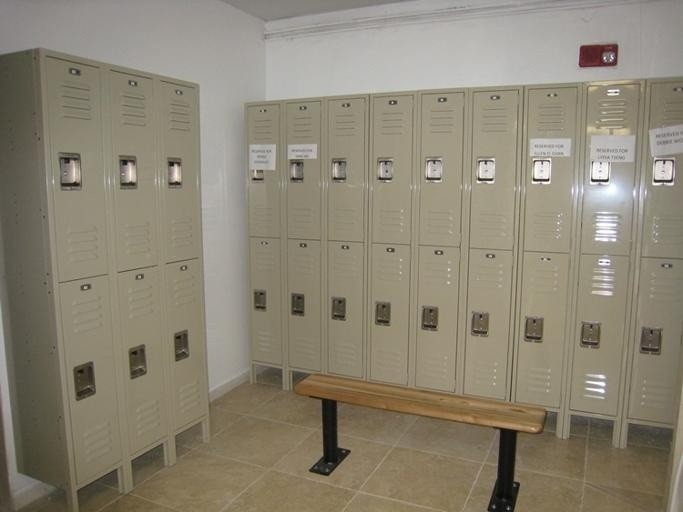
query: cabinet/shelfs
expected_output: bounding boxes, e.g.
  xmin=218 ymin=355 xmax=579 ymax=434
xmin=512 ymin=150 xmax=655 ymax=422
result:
xmin=244 ymin=75 xmax=682 ymax=451
xmin=0 ymin=49 xmax=210 ymax=509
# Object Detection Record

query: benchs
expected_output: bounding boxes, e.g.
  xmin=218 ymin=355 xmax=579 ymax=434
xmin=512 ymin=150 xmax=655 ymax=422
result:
xmin=293 ymin=367 xmax=547 ymax=511
